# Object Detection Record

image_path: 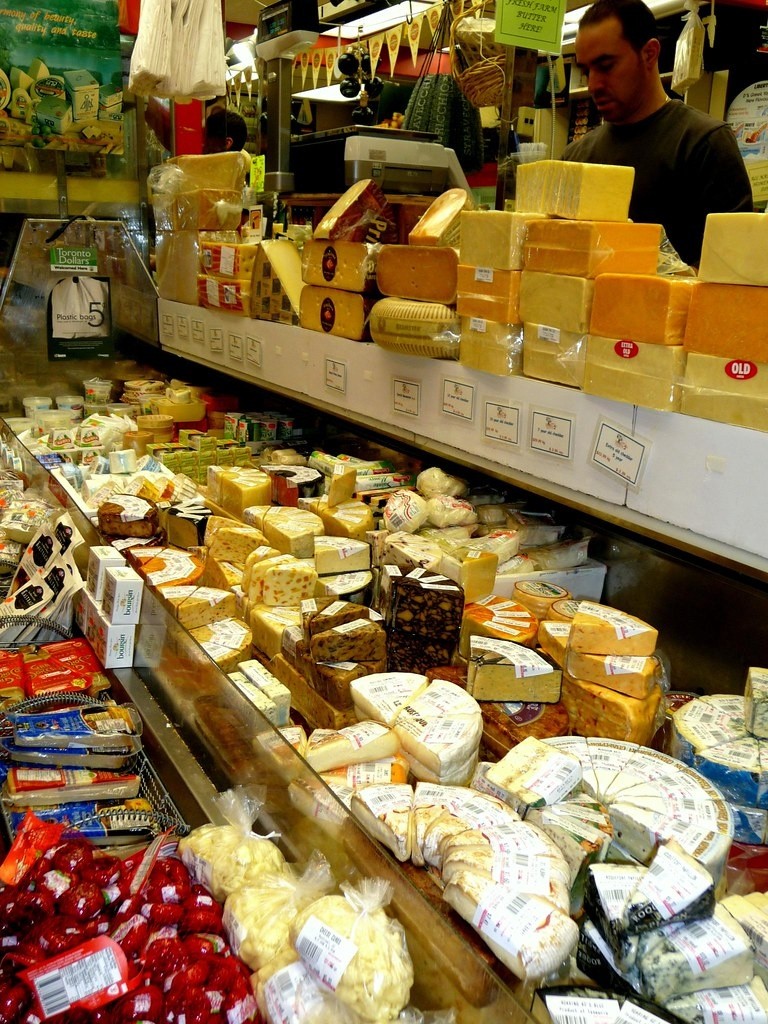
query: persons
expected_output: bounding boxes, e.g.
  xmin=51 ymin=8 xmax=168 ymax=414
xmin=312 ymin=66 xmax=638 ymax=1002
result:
xmin=122 ymin=85 xmax=248 ymax=158
xmin=560 ymin=1 xmax=754 ymax=275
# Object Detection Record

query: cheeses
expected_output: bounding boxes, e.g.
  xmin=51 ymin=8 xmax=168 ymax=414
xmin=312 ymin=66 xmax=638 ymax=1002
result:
xmin=33 ymin=379 xmax=768 ymax=1023
xmin=149 ymin=147 xmax=767 ymax=434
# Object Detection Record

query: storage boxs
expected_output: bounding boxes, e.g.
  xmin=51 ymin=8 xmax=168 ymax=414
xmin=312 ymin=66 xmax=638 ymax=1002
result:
xmin=72 ymin=545 xmax=145 ymax=669
